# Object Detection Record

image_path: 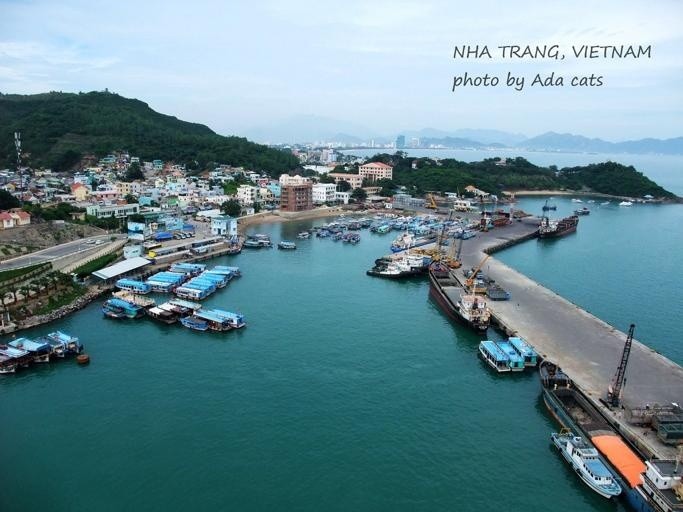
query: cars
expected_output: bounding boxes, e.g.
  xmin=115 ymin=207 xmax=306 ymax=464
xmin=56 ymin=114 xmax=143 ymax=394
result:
xmin=86 ymin=238 xmax=105 ymax=245
xmin=173 ymin=232 xmax=196 ymax=240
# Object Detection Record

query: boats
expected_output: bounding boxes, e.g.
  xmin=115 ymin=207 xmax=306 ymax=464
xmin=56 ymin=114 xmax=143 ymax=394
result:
xmin=539 ymin=358 xmax=683 ymax=512
xmin=571 ymin=198 xmax=633 ymax=207
xmin=465 ymin=196 xmax=518 ymax=205
xmin=243 ymin=232 xmax=273 ymax=249
xmin=277 ymin=240 xmax=296 ymax=250
xmin=0 ymin=330 xmax=84 ymax=375
xmin=366 ymin=250 xmax=434 ymax=279
xmin=478 ymin=336 xmax=538 ymax=374
xmin=542 ymin=200 xmax=557 ymax=210
xmin=101 ymin=262 xmax=247 ymax=333
xmin=537 ymin=215 xmax=579 ymax=239
xmin=573 ymin=208 xmax=590 ymax=214
xmin=295 ymin=210 xmax=480 ymax=252
xmin=429 ymin=260 xmax=492 ymax=332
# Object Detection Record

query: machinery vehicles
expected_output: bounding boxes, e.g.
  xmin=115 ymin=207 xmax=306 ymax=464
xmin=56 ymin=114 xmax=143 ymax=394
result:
xmin=425 ymin=195 xmax=438 ymax=209
xmin=415 ymin=228 xmax=462 ymax=269
xmin=464 ymin=252 xmax=492 ymax=294
xmin=607 ymin=323 xmax=636 ymax=406
